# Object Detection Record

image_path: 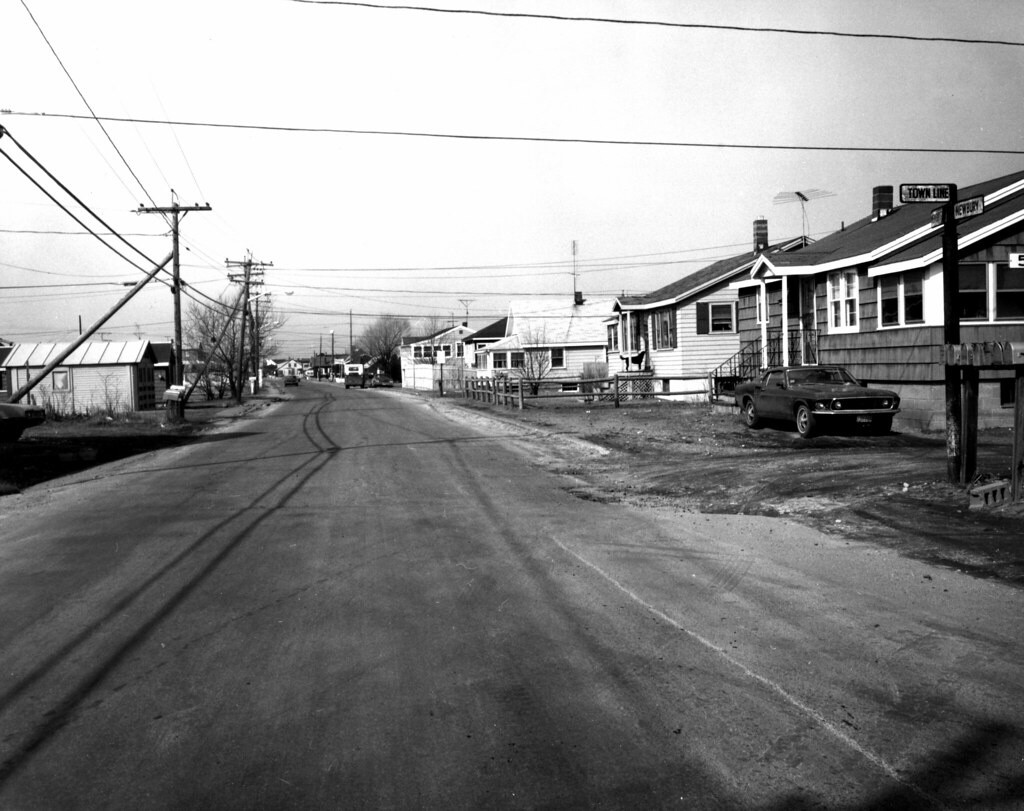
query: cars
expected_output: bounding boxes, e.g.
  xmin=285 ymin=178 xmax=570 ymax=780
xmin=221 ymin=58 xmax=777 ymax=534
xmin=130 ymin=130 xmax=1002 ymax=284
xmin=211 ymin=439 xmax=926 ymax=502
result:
xmin=735 ymin=364 xmax=901 ymax=438
xmin=372 ymin=373 xmax=394 ymax=388
xmin=284 ymin=375 xmax=299 ymax=387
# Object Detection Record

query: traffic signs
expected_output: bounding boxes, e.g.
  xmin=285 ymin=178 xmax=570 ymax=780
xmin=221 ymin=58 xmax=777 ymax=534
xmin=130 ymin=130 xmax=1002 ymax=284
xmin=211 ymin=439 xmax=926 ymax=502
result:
xmin=929 ymin=194 xmax=986 ymax=228
xmin=898 ymin=182 xmax=955 ymax=203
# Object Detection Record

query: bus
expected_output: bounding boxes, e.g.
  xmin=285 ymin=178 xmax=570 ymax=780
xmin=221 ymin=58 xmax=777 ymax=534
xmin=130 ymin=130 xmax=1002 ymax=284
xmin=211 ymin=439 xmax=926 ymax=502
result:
xmin=344 ymin=363 xmax=367 ymax=389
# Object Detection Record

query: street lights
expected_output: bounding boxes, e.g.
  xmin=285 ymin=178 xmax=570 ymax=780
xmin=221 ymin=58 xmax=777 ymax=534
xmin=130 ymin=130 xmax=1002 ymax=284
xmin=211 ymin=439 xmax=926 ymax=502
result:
xmin=237 ymin=290 xmax=294 ymax=405
